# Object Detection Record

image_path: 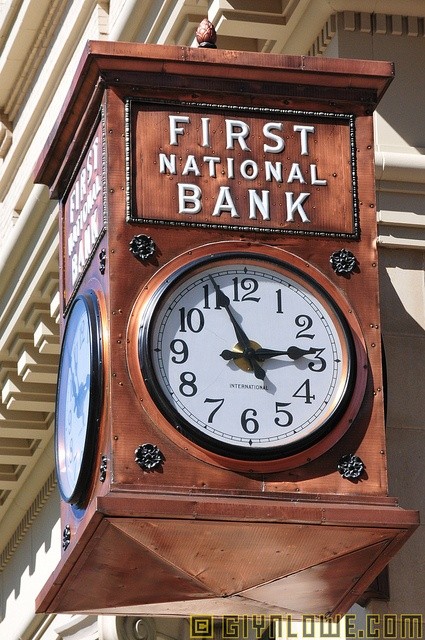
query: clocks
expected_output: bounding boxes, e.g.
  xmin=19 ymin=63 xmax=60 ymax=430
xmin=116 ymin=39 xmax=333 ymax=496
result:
xmin=127 ymin=241 xmax=371 ymax=477
xmin=52 ymin=291 xmax=104 ymax=507
